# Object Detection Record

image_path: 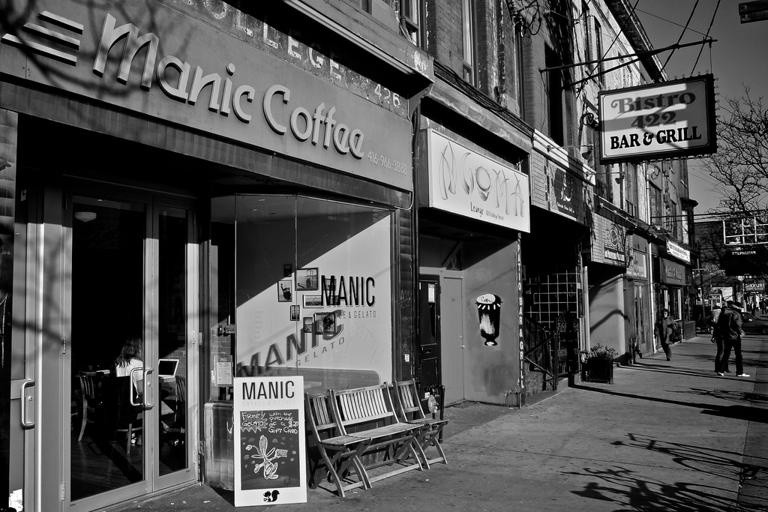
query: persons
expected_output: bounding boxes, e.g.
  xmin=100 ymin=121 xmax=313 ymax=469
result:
xmin=710 ymin=300 xmax=751 ymax=378
xmin=653 ymin=308 xmax=679 ymax=362
xmin=110 ymin=335 xmax=142 ymax=401
xmin=750 ymin=297 xmax=768 ymax=315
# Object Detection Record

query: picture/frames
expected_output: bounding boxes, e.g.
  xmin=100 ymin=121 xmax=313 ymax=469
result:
xmin=303 ymin=294 xmax=325 ymax=307
xmin=314 ymin=312 xmax=337 ymax=335
xmin=290 ymin=305 xmax=300 ymax=320
xmin=295 ymin=268 xmax=319 ymax=290
xmin=277 ymin=280 xmax=292 ymax=303
xmin=303 ymin=317 xmax=312 ymax=333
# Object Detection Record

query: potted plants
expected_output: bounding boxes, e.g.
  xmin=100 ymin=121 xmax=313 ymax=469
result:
xmin=580 ymin=343 xmax=618 ymax=384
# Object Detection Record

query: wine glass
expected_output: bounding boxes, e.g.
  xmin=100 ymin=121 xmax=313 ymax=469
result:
xmin=478 ymin=304 xmax=500 ymax=346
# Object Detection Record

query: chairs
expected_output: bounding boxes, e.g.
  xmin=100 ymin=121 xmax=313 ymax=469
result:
xmin=76 ymin=372 xmax=142 ymax=457
xmin=391 ymin=377 xmax=451 ymax=469
xmin=304 ymin=388 xmax=370 ymax=496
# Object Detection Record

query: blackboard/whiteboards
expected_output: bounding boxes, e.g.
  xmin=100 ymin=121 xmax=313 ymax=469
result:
xmin=240 ymin=409 xmax=300 ymax=490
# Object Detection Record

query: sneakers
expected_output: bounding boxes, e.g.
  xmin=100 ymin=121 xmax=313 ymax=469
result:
xmin=718 ymin=372 xmax=725 ymax=376
xmin=738 ymin=373 xmax=751 ymax=377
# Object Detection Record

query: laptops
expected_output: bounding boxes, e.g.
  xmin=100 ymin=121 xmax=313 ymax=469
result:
xmin=158 ymin=358 xmax=180 ymax=379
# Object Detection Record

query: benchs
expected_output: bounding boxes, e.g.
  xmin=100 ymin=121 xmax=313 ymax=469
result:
xmin=332 ymin=380 xmax=427 ymax=488
